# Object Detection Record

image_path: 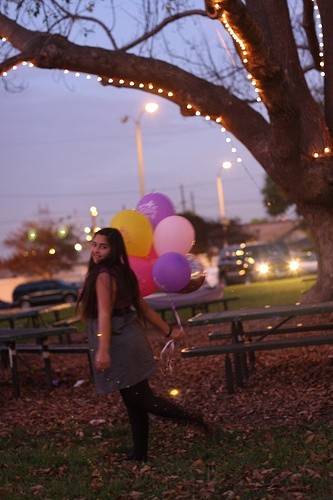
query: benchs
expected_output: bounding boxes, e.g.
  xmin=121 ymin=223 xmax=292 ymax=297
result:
xmin=0 ymin=313 xmax=333 ymax=392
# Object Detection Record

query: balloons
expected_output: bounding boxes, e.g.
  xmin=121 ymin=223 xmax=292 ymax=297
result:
xmin=152 ymin=251 xmax=192 ymax=292
xmin=135 ymin=192 xmax=175 ymax=228
xmin=109 ymin=209 xmax=154 ymax=257
xmin=154 ymin=216 xmax=195 ymax=257
xmin=126 ymin=255 xmax=154 ymax=295
xmin=177 ymin=259 xmax=206 ymax=293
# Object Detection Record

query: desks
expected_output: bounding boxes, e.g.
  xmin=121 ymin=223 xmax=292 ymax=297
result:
xmin=0 ymin=299 xmax=83 ymax=327
xmin=187 ymin=301 xmax=333 ymax=333
xmin=0 ymin=327 xmax=78 ymax=389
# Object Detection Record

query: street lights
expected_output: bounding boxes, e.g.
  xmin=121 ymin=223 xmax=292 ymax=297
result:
xmin=134 ymin=102 xmax=159 ymax=198
xmin=217 ymin=160 xmax=234 ymax=248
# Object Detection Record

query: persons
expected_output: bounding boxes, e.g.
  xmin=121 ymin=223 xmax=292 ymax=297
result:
xmin=74 ymin=228 xmax=221 ymax=462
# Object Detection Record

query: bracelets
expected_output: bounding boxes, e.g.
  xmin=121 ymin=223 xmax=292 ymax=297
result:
xmin=166 ymin=328 xmax=172 ymax=337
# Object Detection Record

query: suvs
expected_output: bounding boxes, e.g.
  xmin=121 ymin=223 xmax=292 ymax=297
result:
xmin=12 ymin=280 xmax=83 ymax=310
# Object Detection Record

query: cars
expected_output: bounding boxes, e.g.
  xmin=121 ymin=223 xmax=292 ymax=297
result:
xmin=218 ymin=241 xmax=299 ymax=288
